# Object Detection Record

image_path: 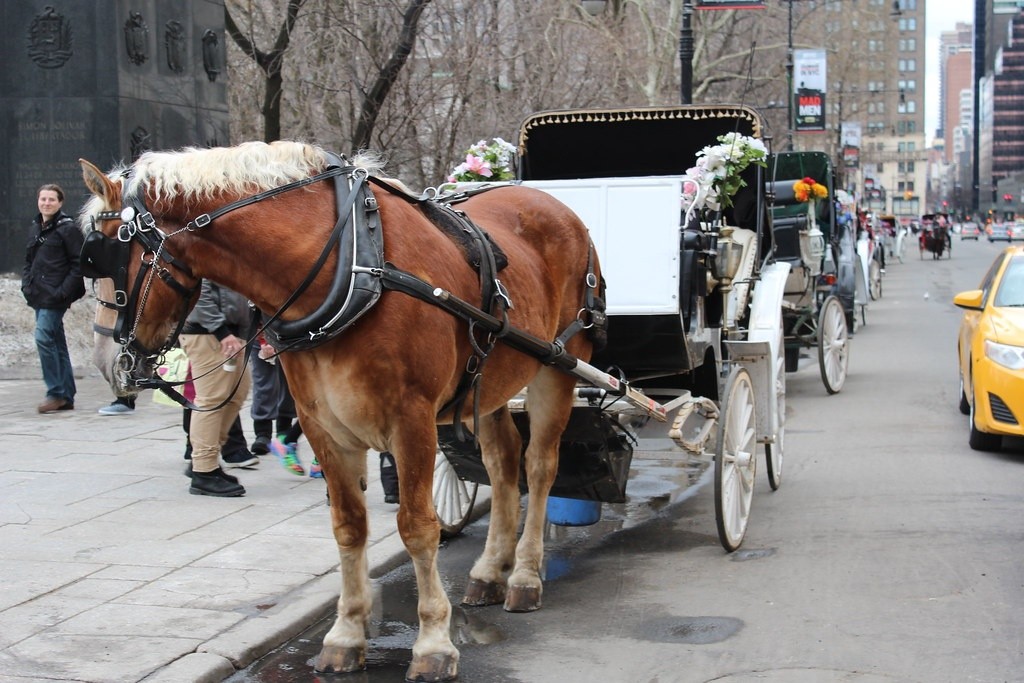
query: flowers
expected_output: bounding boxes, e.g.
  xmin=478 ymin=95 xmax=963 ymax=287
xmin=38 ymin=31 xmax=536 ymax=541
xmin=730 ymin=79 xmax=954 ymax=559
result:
xmin=679 ymin=131 xmax=769 ymax=214
xmin=440 ymin=137 xmax=518 ymax=195
xmin=793 ymin=177 xmax=828 ymax=203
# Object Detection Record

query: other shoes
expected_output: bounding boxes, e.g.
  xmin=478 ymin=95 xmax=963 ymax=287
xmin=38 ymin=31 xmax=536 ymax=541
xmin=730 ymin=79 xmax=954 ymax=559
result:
xmin=40 ymin=398 xmax=73 ymax=412
xmin=224 ymin=448 xmax=259 ymax=468
xmin=251 ymin=436 xmax=271 ymax=453
xmin=184 ymin=444 xmax=192 ymax=463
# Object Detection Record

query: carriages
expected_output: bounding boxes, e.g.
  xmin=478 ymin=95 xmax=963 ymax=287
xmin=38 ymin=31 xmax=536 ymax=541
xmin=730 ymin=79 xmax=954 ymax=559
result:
xmin=79 ymin=104 xmax=792 ymax=683
xmin=762 ymin=151 xmax=849 ymax=393
xmin=919 ymin=214 xmax=951 ymax=260
xmin=814 ymin=189 xmax=907 ymax=333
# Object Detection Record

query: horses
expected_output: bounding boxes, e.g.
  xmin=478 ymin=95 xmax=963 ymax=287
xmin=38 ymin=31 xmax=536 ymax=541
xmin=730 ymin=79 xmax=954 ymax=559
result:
xmin=71 ymin=138 xmax=607 ymax=683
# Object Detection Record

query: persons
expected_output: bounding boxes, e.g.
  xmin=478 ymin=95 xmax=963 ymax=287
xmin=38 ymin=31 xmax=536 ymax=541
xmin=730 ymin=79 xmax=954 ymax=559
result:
xmin=21 ymin=184 xmax=87 ymax=413
xmin=178 ymin=278 xmax=322 ymax=497
xmin=99 ymin=396 xmax=137 ymax=415
xmin=933 ymin=212 xmax=946 ymax=230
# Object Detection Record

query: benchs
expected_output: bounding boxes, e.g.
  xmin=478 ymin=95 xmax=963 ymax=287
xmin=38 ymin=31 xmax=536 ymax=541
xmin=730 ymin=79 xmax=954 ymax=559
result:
xmin=717 ymin=224 xmax=757 ymax=328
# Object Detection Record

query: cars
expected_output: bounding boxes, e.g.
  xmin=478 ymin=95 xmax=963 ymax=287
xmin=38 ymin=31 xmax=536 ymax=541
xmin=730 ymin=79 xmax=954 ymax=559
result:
xmin=1009 ymin=222 xmax=1024 ymax=240
xmin=953 ymin=245 xmax=1024 ymax=450
xmin=987 ymin=224 xmax=1012 ymax=243
xmin=961 ymin=222 xmax=979 ymax=240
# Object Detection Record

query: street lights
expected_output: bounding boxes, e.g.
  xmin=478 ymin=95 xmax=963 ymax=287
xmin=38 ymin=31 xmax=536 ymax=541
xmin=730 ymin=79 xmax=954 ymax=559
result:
xmin=837 ymin=88 xmax=905 ymax=190
xmin=787 ymin=0 xmax=902 ymax=151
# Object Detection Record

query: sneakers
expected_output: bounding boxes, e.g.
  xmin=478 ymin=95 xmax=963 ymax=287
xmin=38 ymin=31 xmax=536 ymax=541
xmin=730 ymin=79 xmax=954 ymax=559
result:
xmin=309 ymin=461 xmax=323 ymax=477
xmin=98 ymin=403 xmax=134 ymax=415
xmin=269 ymin=435 xmax=304 ymax=475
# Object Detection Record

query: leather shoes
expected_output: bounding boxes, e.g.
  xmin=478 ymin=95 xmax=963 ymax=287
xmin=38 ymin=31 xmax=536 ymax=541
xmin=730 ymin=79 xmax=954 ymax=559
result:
xmin=189 ymin=470 xmax=246 ymax=497
xmin=185 ymin=465 xmax=238 ymax=484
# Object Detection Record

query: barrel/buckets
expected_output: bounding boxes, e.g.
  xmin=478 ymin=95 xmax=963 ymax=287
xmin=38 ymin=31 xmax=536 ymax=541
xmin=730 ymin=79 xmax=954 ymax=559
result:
xmin=546 ymin=496 xmax=601 ymax=527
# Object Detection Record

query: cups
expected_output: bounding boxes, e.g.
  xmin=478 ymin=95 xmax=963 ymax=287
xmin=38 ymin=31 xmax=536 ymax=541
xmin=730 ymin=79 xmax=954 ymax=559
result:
xmin=223 ymin=352 xmax=236 ymax=371
